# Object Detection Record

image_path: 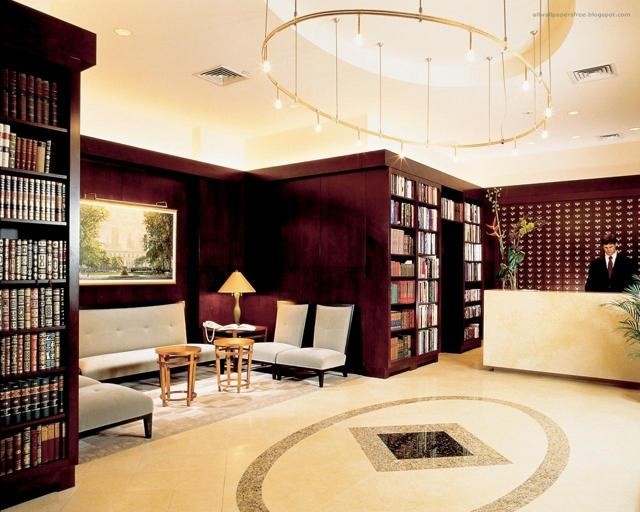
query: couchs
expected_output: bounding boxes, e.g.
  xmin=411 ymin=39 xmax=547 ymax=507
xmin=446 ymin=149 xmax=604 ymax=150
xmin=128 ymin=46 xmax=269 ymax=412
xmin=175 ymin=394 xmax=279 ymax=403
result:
xmin=79 ymin=301 xmax=226 ymax=384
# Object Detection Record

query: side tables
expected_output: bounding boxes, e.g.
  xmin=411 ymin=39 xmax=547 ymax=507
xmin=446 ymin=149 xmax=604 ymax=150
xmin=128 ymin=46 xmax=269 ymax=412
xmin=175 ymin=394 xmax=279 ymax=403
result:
xmin=202 ymin=325 xmax=268 ymax=345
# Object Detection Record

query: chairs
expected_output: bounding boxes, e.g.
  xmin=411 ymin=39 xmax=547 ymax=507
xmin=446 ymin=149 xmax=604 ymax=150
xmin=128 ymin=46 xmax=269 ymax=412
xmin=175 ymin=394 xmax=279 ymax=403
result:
xmin=234 ymin=304 xmax=309 ymax=380
xmin=276 ymin=304 xmax=355 ymax=387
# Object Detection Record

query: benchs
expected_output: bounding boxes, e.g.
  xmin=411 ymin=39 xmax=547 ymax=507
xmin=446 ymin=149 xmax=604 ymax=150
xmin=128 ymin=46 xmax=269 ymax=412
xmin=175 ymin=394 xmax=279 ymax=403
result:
xmin=79 ymin=375 xmax=154 ymax=439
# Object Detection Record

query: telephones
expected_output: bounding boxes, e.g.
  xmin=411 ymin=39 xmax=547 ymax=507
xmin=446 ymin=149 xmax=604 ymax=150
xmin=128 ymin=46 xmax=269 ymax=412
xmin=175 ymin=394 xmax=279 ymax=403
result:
xmin=202 ymin=320 xmax=223 ymax=329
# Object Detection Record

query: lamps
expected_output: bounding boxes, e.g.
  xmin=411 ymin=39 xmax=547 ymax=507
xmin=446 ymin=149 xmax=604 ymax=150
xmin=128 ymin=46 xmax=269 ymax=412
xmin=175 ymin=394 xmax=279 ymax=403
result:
xmin=218 ymin=272 xmax=257 ymax=326
xmin=260 ymin=0 xmax=551 ymax=158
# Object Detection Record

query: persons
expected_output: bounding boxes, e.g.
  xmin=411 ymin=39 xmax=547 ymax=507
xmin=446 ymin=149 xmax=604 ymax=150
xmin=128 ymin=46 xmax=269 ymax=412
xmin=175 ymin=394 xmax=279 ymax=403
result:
xmin=585 ymin=238 xmax=636 ymax=293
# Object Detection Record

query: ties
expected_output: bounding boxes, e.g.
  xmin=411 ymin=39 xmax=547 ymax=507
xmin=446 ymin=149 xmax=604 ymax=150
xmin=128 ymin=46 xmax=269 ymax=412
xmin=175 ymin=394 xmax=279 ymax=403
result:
xmin=608 ymin=256 xmax=613 ymax=276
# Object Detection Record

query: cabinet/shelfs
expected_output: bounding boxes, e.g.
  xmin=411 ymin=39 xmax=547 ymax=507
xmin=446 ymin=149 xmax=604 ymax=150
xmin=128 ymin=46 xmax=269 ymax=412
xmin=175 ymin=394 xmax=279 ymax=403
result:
xmin=366 ymin=168 xmax=485 ymax=379
xmin=0 ymin=0 xmax=96 ymax=511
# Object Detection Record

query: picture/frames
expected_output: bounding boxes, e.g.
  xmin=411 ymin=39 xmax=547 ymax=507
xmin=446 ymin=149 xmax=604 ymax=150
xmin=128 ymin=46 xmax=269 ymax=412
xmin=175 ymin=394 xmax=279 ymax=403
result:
xmin=80 ymin=199 xmax=178 ymax=286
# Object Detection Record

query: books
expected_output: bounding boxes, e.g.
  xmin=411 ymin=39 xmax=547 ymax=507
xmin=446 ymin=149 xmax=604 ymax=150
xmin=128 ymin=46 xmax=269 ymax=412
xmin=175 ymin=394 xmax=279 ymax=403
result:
xmin=389 ymin=174 xmax=482 ymax=365
xmin=0 ymin=66 xmax=68 ymax=476
